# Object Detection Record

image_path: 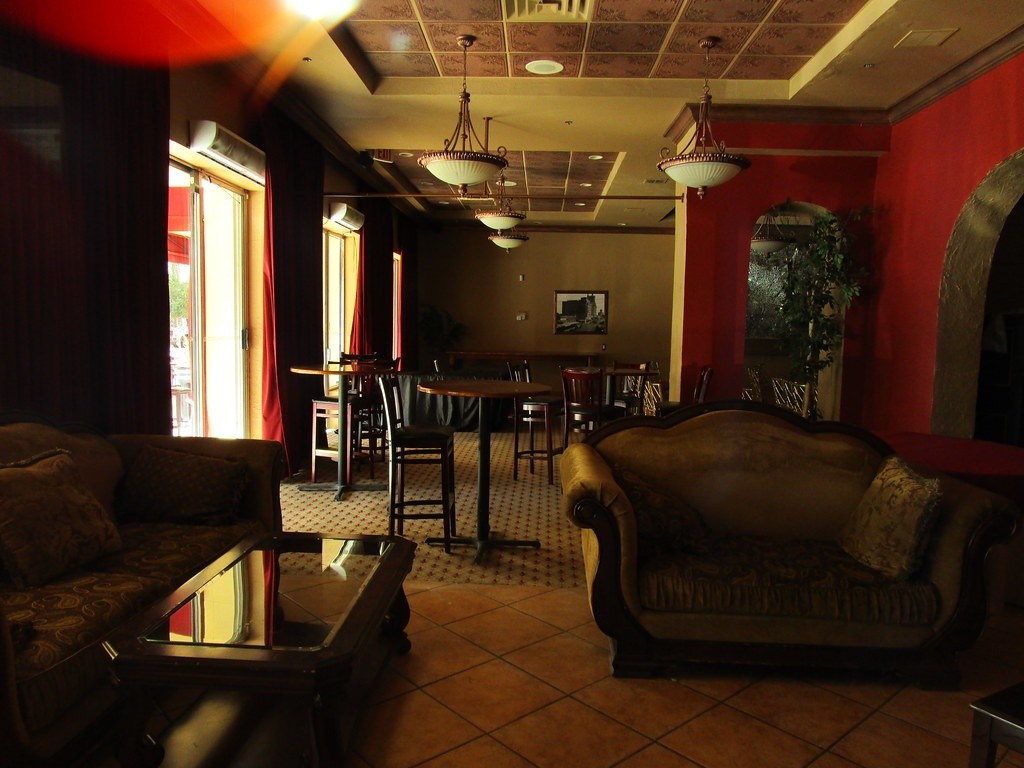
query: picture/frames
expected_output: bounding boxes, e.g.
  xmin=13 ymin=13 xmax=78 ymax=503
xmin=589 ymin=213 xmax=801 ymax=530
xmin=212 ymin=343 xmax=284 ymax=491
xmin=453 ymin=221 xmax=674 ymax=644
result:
xmin=553 ymin=289 xmax=610 ymax=336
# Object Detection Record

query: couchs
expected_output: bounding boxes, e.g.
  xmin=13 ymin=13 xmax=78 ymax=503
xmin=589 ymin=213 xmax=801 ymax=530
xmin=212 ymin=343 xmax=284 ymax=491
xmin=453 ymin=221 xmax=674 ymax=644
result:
xmin=0 ymin=425 xmax=283 ymax=768
xmin=560 ymin=393 xmax=1022 ymax=692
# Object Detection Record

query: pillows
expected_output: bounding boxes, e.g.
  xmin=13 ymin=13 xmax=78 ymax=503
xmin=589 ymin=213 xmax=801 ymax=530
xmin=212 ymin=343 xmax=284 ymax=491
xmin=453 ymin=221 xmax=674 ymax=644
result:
xmin=117 ymin=443 xmax=246 ymax=530
xmin=1 ymin=449 xmax=125 ymax=585
xmin=835 ymin=453 xmax=947 ymax=584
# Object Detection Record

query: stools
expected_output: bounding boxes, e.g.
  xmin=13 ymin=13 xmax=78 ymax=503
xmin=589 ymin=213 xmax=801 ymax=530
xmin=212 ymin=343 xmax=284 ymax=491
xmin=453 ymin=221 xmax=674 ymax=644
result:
xmin=968 ymin=683 xmax=1024 ymax=768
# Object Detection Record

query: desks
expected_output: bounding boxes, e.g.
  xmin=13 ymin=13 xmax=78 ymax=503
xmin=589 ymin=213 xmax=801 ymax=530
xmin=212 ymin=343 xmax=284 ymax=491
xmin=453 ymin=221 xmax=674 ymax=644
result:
xmin=443 ymin=349 xmax=601 ymax=385
xmin=601 ymin=368 xmax=660 ymax=417
xmin=288 ymin=363 xmax=389 ymax=501
xmin=414 ymin=377 xmax=541 ymax=565
xmin=885 ymin=432 xmax=1023 ymax=490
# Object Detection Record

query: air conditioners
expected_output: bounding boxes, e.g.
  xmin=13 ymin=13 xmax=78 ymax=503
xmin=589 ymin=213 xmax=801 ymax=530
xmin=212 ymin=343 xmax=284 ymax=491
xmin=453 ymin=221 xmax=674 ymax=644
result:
xmin=329 ymin=202 xmax=365 ymax=231
xmin=188 ymin=119 xmax=269 ymax=188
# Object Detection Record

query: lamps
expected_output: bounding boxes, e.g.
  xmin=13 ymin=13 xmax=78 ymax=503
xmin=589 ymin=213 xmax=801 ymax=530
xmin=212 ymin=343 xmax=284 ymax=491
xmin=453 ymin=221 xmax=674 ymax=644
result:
xmin=750 ymin=211 xmax=796 ymax=258
xmin=487 ymin=198 xmax=529 ymax=256
xmin=416 ymin=34 xmax=509 ymax=199
xmin=654 ymin=35 xmax=752 ymax=202
xmin=474 ymin=151 xmax=528 ymax=237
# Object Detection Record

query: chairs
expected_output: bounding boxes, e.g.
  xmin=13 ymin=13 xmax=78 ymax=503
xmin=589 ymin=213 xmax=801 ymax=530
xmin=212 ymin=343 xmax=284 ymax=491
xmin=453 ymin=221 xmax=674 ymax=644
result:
xmin=328 ymin=354 xmax=402 ymax=466
xmin=309 ymin=350 xmax=383 ymax=484
xmin=558 ymin=364 xmax=627 ymax=455
xmin=377 ymin=373 xmax=459 ymax=554
xmin=655 ymin=366 xmax=713 ymax=418
xmin=505 ymin=359 xmax=568 ymax=485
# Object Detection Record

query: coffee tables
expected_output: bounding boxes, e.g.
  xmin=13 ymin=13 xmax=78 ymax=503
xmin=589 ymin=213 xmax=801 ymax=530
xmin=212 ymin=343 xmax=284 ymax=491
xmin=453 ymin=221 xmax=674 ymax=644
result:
xmin=99 ymin=527 xmax=419 ymax=768
